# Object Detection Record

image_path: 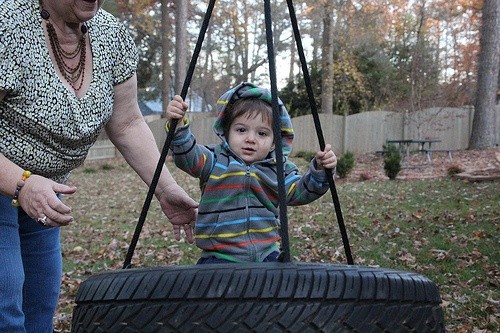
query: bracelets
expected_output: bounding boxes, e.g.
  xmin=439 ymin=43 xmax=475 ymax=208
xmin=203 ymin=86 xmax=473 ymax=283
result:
xmin=11 ymin=170 xmax=31 ymax=207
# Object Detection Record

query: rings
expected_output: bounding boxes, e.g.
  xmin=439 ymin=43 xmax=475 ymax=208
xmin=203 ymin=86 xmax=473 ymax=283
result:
xmin=37 ymin=216 xmax=48 ymax=226
xmin=182 ymin=224 xmax=191 ymax=226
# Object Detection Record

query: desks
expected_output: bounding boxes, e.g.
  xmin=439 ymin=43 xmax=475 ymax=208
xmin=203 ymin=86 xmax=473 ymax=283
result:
xmin=386 ymin=139 xmax=441 ymax=169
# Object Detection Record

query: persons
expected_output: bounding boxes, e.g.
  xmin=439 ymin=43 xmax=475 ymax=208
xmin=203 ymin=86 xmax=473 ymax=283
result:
xmin=0 ymin=0 xmax=200 ymax=333
xmin=165 ymin=82 xmax=338 ymax=265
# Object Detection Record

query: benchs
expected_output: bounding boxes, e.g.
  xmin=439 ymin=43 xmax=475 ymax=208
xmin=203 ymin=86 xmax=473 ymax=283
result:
xmin=376 ymin=148 xmax=459 ymax=165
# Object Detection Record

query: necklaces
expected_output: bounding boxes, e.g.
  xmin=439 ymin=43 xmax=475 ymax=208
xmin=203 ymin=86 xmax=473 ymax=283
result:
xmin=39 ymin=0 xmax=87 ymax=91
